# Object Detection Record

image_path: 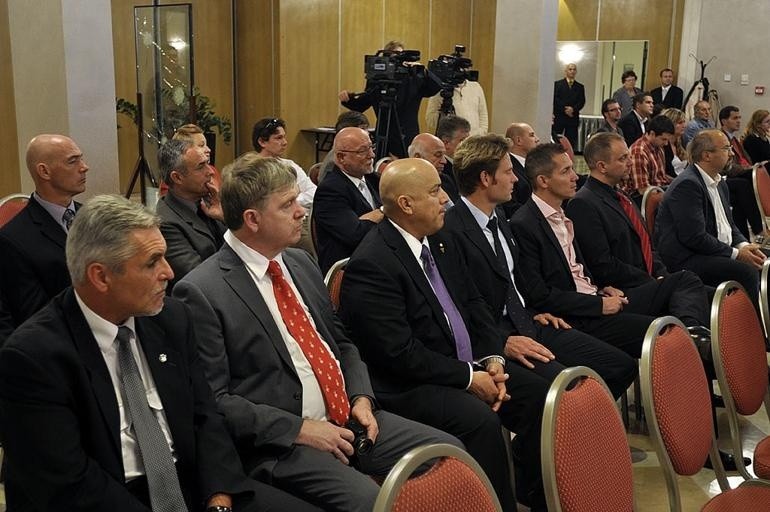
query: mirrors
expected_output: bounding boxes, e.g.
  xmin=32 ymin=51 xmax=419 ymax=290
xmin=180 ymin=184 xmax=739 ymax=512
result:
xmin=552 ymin=37 xmax=651 ymax=124
xmin=122 ymin=3 xmax=202 ymax=210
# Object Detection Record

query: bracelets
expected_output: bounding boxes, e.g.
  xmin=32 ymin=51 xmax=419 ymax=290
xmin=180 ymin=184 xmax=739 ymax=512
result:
xmin=483 ymin=357 xmax=505 ymax=367
xmin=207 ymin=505 xmax=231 ymax=512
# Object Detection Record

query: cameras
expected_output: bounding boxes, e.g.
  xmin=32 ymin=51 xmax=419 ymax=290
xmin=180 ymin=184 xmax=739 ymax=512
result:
xmin=344 ymin=420 xmax=374 ymax=457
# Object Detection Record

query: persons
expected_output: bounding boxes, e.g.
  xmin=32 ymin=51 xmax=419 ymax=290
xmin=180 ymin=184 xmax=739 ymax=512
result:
xmin=339 ymin=158 xmax=552 ymax=512
xmin=253 ymin=117 xmax=320 ymax=264
xmin=336 ymin=42 xmax=445 ymax=163
xmin=566 ymin=133 xmax=724 ymax=409
xmin=1 ymin=194 xmax=326 ymax=512
xmin=155 ymin=140 xmax=228 ymax=299
xmin=613 ymin=71 xmax=644 ymax=120
xmin=159 ymin=123 xmax=222 ymax=206
xmin=618 ymin=116 xmax=675 ymax=212
xmin=171 ymin=152 xmax=465 ymax=512
xmin=680 ymin=101 xmax=717 ymax=153
xmin=551 ymin=64 xmax=586 ymax=153
xmin=649 ymin=69 xmax=683 ymax=119
xmin=429 ymin=133 xmax=633 ymax=404
xmin=660 ymin=108 xmax=689 ymax=178
xmin=316 ymin=109 xmax=370 ymax=188
xmin=500 ymin=123 xmax=540 ymax=218
xmin=652 ymin=129 xmax=769 ymax=354
xmin=619 ymin=93 xmax=654 ymax=150
xmin=740 ymin=109 xmax=769 ymax=174
xmin=588 ymin=98 xmax=623 ymax=138
xmin=508 ymin=144 xmax=751 ymax=470
xmin=0 ymin=133 xmax=88 ymax=331
xmin=313 ymin=128 xmax=385 ymax=279
xmin=424 ymin=69 xmax=489 ymax=139
xmin=717 ymin=106 xmax=770 ymax=243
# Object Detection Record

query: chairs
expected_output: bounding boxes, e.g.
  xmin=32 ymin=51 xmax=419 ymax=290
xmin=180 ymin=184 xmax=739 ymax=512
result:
xmin=0 ymin=191 xmax=41 ymax=238
xmin=368 ymin=442 xmax=508 ymax=511
xmin=758 ymin=260 xmax=770 ymax=338
xmin=748 ymin=160 xmax=770 ymax=238
xmin=317 ymin=254 xmax=357 ymax=329
xmin=633 ymin=182 xmax=666 ymax=251
xmin=710 ymin=280 xmax=770 ymax=484
xmin=556 ymin=134 xmax=574 ymax=160
xmin=306 ymin=162 xmax=323 ymax=185
xmin=537 ymin=363 xmax=636 ymax=512
xmin=373 ymin=157 xmax=395 ymax=176
xmin=639 ymin=315 xmax=770 ymax=511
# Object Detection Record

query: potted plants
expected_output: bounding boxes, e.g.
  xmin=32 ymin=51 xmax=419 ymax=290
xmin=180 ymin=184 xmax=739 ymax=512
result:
xmin=191 ymin=88 xmax=232 ymax=172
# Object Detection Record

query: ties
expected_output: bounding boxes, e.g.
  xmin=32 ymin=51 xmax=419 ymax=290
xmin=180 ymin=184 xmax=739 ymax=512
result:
xmin=614 ymin=191 xmax=652 ymax=275
xmin=195 ymin=201 xmax=225 ymax=251
xmin=421 ymin=245 xmax=475 ymax=360
xmin=268 ymin=260 xmax=353 ymax=424
xmin=65 ymin=210 xmax=76 ymax=230
xmin=569 ymin=81 xmax=573 ymax=89
xmin=360 ymin=182 xmax=377 ymax=208
xmin=486 ymin=217 xmax=537 ymax=340
xmin=117 ymin=326 xmax=190 ymax=512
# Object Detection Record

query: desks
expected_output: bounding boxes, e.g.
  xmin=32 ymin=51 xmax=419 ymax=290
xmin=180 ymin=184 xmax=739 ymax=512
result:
xmin=301 ymin=123 xmax=385 ymax=170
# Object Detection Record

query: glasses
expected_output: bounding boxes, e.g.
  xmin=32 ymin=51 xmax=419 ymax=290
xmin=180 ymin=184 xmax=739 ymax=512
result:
xmin=265 ymin=119 xmax=279 ymax=129
xmin=339 ymin=145 xmax=377 ymax=155
